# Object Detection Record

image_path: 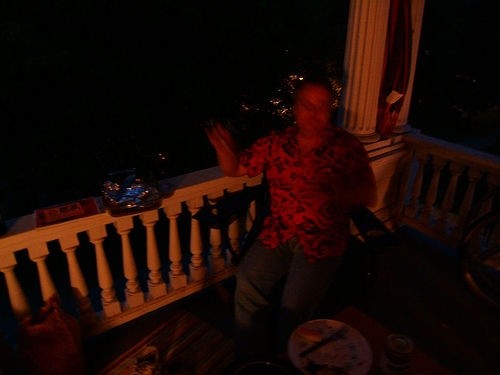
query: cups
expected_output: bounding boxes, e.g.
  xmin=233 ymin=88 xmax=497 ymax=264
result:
xmin=234 ymin=361 xmax=287 ymax=375
xmin=386 ymin=334 xmax=413 ymax=369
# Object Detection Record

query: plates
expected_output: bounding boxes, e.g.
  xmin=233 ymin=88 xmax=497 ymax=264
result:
xmin=288 ymin=319 xmax=373 ymax=375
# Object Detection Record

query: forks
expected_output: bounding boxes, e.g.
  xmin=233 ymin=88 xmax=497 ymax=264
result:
xmin=299 ymin=325 xmax=352 ymax=358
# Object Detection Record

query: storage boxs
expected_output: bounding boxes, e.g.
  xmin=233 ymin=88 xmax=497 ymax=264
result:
xmin=99 ymin=168 xmax=161 ymax=216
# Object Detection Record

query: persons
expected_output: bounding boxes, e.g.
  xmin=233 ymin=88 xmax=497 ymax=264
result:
xmin=208 ymin=83 xmax=377 ymax=330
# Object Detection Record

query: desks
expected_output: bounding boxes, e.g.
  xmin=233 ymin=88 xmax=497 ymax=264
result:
xmin=232 ymin=301 xmax=455 ymax=375
